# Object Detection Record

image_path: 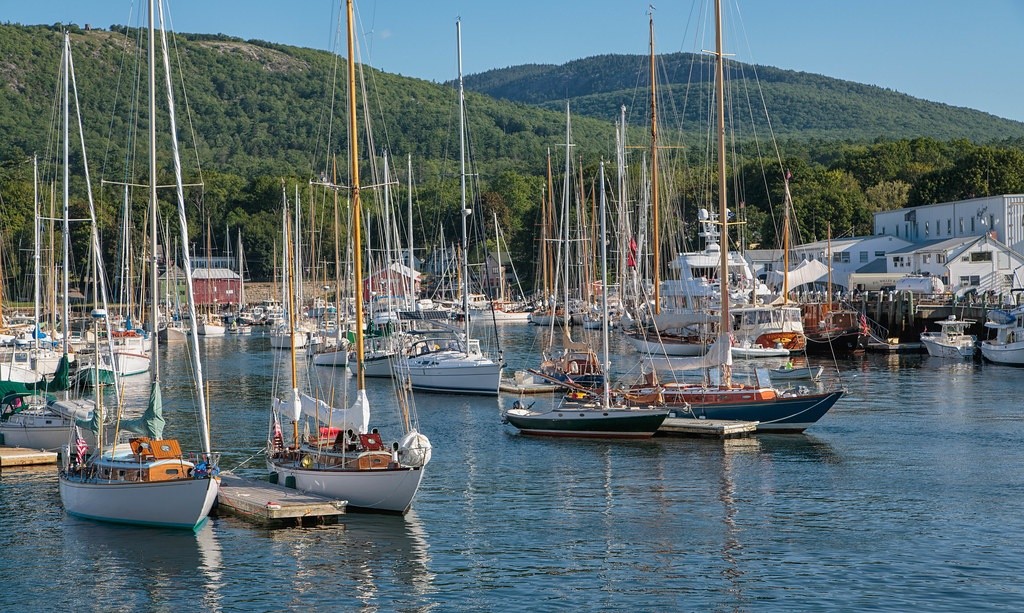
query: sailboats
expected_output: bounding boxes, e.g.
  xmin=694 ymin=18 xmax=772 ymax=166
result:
xmin=0 ymin=1 xmax=1024 ymax=531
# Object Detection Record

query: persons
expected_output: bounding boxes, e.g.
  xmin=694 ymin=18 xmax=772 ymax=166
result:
xmin=428 ymin=340 xmax=435 ymax=351
xmin=232 ymin=320 xmax=236 ymax=327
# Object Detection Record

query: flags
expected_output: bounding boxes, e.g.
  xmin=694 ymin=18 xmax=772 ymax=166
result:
xmin=272 ymin=410 xmax=284 ymax=451
xmin=75 ymin=426 xmax=89 ymax=465
xmin=859 ymin=306 xmax=868 ymax=334
